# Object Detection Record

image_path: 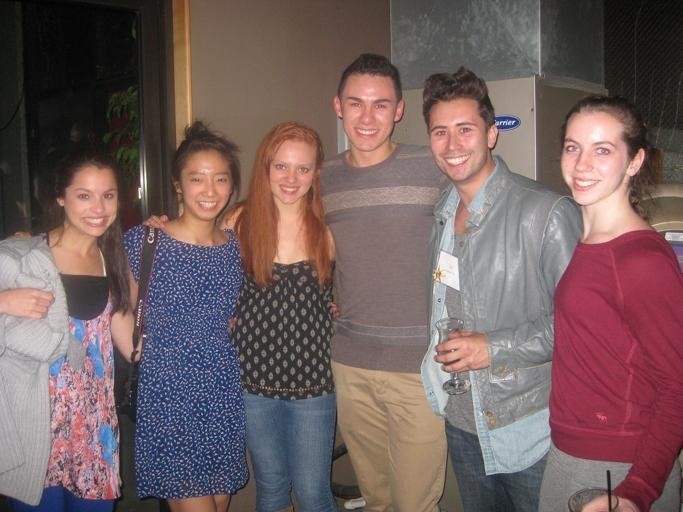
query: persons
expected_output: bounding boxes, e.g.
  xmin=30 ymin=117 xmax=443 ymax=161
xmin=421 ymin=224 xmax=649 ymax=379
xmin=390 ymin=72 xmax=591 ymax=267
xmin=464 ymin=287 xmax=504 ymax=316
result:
xmin=419 ymin=68 xmax=585 ymax=511
xmin=144 ymin=124 xmax=339 ymax=512
xmin=122 ymin=123 xmax=244 ymax=511
xmin=318 ymin=53 xmax=454 ymax=512
xmin=536 ymin=96 xmax=683 ymax=511
xmin=0 ymin=148 xmax=144 ymax=512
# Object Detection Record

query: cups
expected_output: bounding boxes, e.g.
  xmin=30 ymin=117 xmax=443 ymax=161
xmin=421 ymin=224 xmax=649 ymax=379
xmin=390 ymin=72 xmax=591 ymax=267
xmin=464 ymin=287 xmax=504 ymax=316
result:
xmin=569 ymin=489 xmax=617 ymax=511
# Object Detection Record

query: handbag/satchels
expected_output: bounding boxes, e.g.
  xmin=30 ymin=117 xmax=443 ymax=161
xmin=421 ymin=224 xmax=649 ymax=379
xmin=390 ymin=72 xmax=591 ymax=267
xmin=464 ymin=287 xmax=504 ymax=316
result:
xmin=112 ymin=224 xmax=160 ymax=420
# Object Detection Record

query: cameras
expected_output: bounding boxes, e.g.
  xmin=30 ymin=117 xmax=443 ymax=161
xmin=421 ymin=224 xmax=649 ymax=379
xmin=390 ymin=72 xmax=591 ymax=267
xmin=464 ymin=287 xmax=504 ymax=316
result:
xmin=120 ymin=363 xmax=137 ymax=420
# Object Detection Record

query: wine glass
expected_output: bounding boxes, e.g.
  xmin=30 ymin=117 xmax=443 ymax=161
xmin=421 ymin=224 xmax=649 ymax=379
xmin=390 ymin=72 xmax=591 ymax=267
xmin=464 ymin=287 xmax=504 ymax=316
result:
xmin=438 ymin=320 xmax=469 ymax=397
